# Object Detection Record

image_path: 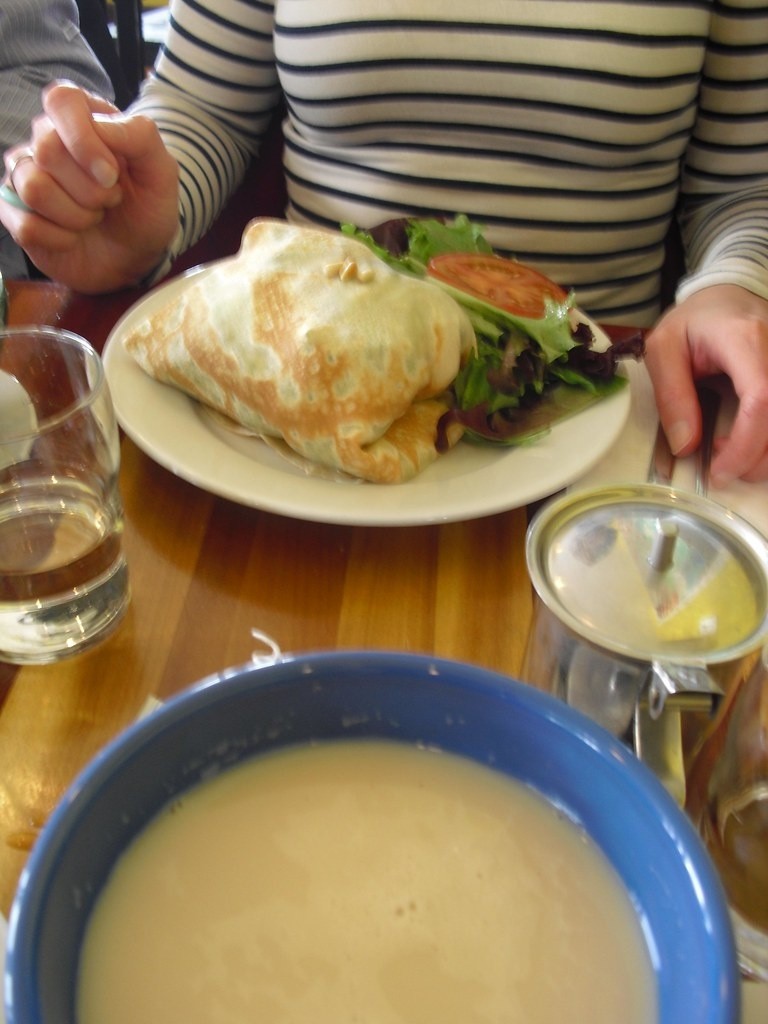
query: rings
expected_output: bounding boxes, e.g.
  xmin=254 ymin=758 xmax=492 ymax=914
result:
xmin=8 ymin=150 xmax=33 ymax=191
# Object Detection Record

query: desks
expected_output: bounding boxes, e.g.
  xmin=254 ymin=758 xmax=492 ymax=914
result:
xmin=0 ymin=278 xmax=768 ymax=1024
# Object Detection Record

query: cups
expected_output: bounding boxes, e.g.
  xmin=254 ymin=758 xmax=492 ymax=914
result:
xmin=518 ymin=480 xmax=768 ymax=820
xmin=0 ymin=322 xmax=135 ymax=667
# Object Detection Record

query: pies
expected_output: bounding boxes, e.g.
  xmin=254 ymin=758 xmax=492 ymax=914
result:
xmin=119 ymin=219 xmax=478 ymax=483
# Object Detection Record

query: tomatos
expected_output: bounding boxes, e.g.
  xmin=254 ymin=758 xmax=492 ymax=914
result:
xmin=424 ymin=251 xmax=572 ymax=319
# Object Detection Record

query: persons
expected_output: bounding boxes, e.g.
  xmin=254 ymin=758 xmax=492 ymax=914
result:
xmin=0 ymin=0 xmax=768 ymax=490
xmin=0 ymin=0 xmax=115 ymax=281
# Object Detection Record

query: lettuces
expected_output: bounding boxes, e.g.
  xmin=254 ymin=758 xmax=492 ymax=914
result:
xmin=342 ymin=218 xmax=648 ymax=443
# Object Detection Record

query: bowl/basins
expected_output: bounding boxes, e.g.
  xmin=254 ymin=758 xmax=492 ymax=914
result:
xmin=0 ymin=648 xmax=740 ymax=1024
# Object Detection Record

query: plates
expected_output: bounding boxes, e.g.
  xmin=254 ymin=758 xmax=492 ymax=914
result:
xmin=101 ymin=254 xmax=630 ymax=528
